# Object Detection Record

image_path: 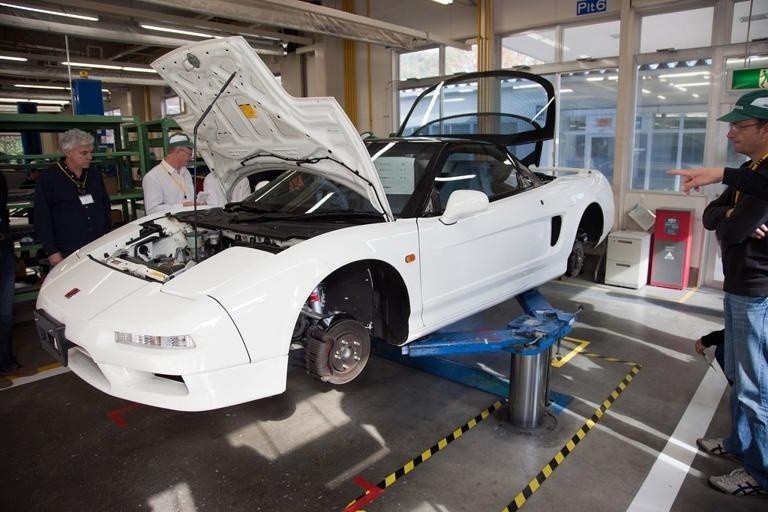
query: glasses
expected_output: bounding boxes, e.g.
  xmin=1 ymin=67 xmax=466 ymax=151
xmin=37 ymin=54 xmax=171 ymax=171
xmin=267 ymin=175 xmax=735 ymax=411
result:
xmin=729 ymin=123 xmax=765 ymax=132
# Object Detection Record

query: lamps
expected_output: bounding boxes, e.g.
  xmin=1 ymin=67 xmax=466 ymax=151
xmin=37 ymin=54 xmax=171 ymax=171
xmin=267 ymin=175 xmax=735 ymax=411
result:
xmin=60 ymin=61 xmax=160 ymax=75
xmin=137 ymin=23 xmax=237 ymax=41
xmin=1 ymin=53 xmax=29 ymax=65
xmin=0 ymin=98 xmax=71 ymax=107
xmin=0 ymin=2 xmax=102 ymax=23
xmin=250 ymin=42 xmax=290 ymax=59
xmin=12 ymin=83 xmax=113 ymax=96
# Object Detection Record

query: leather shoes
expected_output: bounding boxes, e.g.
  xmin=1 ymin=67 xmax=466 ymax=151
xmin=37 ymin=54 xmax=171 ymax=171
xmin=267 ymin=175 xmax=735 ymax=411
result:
xmin=1 ymin=362 xmax=37 ymax=376
xmin=0 ymin=379 xmax=13 ymax=389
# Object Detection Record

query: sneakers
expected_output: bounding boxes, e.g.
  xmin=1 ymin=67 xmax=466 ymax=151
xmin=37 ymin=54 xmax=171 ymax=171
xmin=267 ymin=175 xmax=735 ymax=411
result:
xmin=696 ymin=436 xmax=742 ymax=463
xmin=707 ymin=467 xmax=765 ymax=497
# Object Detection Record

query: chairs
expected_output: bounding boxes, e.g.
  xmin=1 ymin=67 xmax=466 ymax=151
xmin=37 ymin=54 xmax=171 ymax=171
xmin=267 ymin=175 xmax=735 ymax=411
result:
xmin=437 ymin=160 xmax=506 ymax=206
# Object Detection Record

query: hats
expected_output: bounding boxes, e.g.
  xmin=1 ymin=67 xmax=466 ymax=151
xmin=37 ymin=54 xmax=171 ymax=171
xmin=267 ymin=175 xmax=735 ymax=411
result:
xmin=716 ymin=89 xmax=768 ymax=124
xmin=168 ymin=132 xmax=196 ymax=148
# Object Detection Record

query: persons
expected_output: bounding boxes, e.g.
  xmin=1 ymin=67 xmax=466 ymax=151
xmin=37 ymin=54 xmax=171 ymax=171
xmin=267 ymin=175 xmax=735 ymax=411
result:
xmin=19 ymin=168 xmax=41 ymax=241
xmin=665 ymin=166 xmax=768 ymax=200
xmin=0 ymin=172 xmax=38 ymax=390
xmin=33 ymin=127 xmax=114 ymax=268
xmin=695 ymin=329 xmax=734 ymax=387
xmin=204 ymin=172 xmax=250 ymax=207
xmin=142 ymin=134 xmax=207 ymax=214
xmin=698 ymin=89 xmax=768 ymax=501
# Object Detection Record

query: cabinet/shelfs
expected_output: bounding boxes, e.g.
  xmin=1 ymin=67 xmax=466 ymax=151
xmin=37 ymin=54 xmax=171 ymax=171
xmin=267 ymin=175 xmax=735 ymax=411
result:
xmin=124 ymin=115 xmax=210 ymax=215
xmin=604 ymin=228 xmax=651 ymax=290
xmin=0 ymin=112 xmax=148 ymax=304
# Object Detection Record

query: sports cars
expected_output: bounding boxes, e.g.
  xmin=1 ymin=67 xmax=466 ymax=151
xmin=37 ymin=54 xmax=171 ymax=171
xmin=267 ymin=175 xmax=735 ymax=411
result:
xmin=35 ymin=37 xmax=615 ymax=412
